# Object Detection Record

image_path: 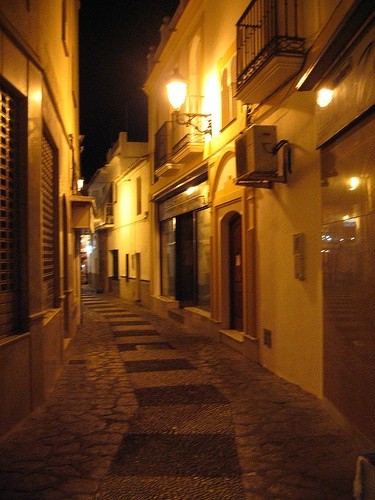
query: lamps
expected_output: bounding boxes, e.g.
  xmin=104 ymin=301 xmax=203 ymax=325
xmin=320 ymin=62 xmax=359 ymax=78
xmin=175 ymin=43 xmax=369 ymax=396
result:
xmin=165 ymin=69 xmax=213 ymax=135
